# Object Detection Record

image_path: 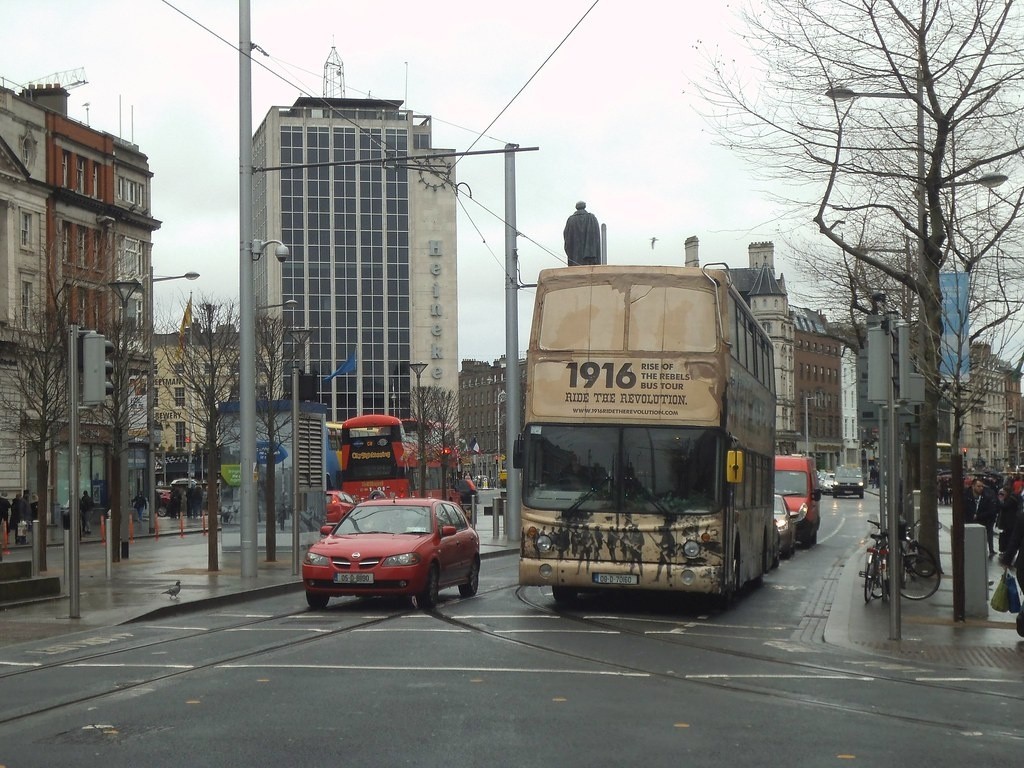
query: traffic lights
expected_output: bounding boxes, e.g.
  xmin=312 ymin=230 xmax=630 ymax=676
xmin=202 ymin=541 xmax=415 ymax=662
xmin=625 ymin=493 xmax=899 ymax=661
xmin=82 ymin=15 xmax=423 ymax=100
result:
xmin=83 ymin=330 xmax=115 ymax=404
xmin=963 ymin=448 xmax=966 ymax=456
xmin=890 ymin=321 xmax=912 ymax=403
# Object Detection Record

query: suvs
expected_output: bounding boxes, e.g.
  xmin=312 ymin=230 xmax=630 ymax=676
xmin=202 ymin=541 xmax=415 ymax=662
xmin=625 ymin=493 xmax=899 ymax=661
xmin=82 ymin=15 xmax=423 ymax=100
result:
xmin=327 ymin=489 xmax=355 ymax=522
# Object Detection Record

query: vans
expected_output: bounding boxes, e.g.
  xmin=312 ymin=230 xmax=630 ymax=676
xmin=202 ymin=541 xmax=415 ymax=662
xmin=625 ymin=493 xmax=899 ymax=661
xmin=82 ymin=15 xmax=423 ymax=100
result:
xmin=832 ymin=465 xmax=866 ymax=500
xmin=775 ymin=454 xmax=821 ymax=549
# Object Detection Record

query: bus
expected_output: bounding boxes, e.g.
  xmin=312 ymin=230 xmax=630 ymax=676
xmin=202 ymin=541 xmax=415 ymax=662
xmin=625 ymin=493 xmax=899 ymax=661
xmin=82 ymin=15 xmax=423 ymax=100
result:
xmin=341 ymin=416 xmax=463 ymax=504
xmin=936 ymin=443 xmax=953 ymax=469
xmin=464 ymin=453 xmax=507 ymax=481
xmin=512 ymin=265 xmax=776 ymax=606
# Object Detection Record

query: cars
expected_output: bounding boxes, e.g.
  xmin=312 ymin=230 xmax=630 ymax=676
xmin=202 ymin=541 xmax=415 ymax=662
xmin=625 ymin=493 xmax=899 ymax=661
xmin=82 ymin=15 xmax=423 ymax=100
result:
xmin=473 ymin=475 xmax=491 ymax=485
xmin=775 ymin=494 xmax=798 ymax=557
xmin=937 ymin=470 xmax=965 ymax=484
xmin=155 ymin=487 xmax=173 ymax=517
xmin=303 ymin=498 xmax=479 ymax=610
xmin=818 ymin=471 xmax=834 ymax=494
xmin=458 ymin=478 xmax=478 ymax=504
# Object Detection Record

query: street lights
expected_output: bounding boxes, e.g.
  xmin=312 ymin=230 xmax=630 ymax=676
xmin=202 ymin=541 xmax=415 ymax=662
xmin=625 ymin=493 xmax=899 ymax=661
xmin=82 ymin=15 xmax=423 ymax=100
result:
xmin=411 ymin=361 xmax=429 ymax=496
xmin=975 ymin=422 xmax=983 ymax=459
xmin=145 ymin=273 xmax=200 ymax=532
xmin=110 ymin=278 xmax=142 ymax=559
xmin=497 ymin=391 xmax=507 ymax=485
xmin=805 ymin=397 xmax=817 ymax=456
xmin=1007 ymin=415 xmax=1017 ymax=466
xmin=826 ymin=88 xmax=1007 ymax=637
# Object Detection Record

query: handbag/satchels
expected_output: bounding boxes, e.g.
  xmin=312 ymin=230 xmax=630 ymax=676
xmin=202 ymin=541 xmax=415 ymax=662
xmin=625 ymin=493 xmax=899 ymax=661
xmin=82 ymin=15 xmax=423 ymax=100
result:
xmin=1005 ymin=572 xmax=1023 ymax=613
xmin=990 ymin=573 xmax=1009 ymax=613
xmin=134 ymin=502 xmax=139 ymax=509
xmin=17 ymin=522 xmax=27 ymax=536
xmin=999 ymin=533 xmax=1007 ymax=552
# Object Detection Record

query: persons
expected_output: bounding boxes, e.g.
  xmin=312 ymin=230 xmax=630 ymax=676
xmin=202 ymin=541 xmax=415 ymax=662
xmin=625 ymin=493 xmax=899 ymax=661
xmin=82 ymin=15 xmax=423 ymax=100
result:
xmin=171 ymin=485 xmax=208 ymax=519
xmin=80 ymin=491 xmax=95 ymax=535
xmin=939 ymin=473 xmax=1024 ymax=638
xmin=131 ymin=491 xmax=147 ymax=528
xmin=870 ymin=466 xmax=876 ymax=489
xmin=563 ymin=201 xmax=601 ymax=267
xmin=0 ymin=491 xmax=32 ymax=545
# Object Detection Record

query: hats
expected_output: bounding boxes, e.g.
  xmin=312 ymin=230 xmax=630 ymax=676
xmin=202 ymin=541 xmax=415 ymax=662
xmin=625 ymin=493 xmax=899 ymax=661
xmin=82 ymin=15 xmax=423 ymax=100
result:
xmin=1013 ymin=481 xmax=1024 ymax=494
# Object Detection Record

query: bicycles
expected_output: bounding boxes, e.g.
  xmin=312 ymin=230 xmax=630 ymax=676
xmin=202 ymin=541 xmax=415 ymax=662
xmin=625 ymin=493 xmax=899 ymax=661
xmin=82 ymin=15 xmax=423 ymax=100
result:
xmin=859 ymin=518 xmax=946 ymax=603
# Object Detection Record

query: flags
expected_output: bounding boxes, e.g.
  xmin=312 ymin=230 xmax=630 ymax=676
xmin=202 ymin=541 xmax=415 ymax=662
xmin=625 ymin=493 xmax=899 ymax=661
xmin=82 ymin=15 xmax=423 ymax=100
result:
xmin=469 ymin=439 xmax=483 ymax=456
xmin=173 ymin=296 xmax=192 ymax=364
xmin=323 ymin=345 xmax=356 ymax=384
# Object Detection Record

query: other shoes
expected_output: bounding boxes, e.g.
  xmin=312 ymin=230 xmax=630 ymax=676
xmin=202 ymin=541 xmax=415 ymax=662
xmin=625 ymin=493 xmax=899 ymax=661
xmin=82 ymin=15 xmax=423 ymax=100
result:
xmin=16 ymin=542 xmax=21 ymax=545
xmin=21 ymin=541 xmax=29 ymax=545
xmin=990 ymin=551 xmax=997 ymax=555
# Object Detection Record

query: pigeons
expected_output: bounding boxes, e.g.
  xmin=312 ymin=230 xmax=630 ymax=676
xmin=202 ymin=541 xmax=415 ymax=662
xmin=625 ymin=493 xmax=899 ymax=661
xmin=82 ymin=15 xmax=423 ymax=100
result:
xmin=161 ymin=581 xmax=181 ymax=599
